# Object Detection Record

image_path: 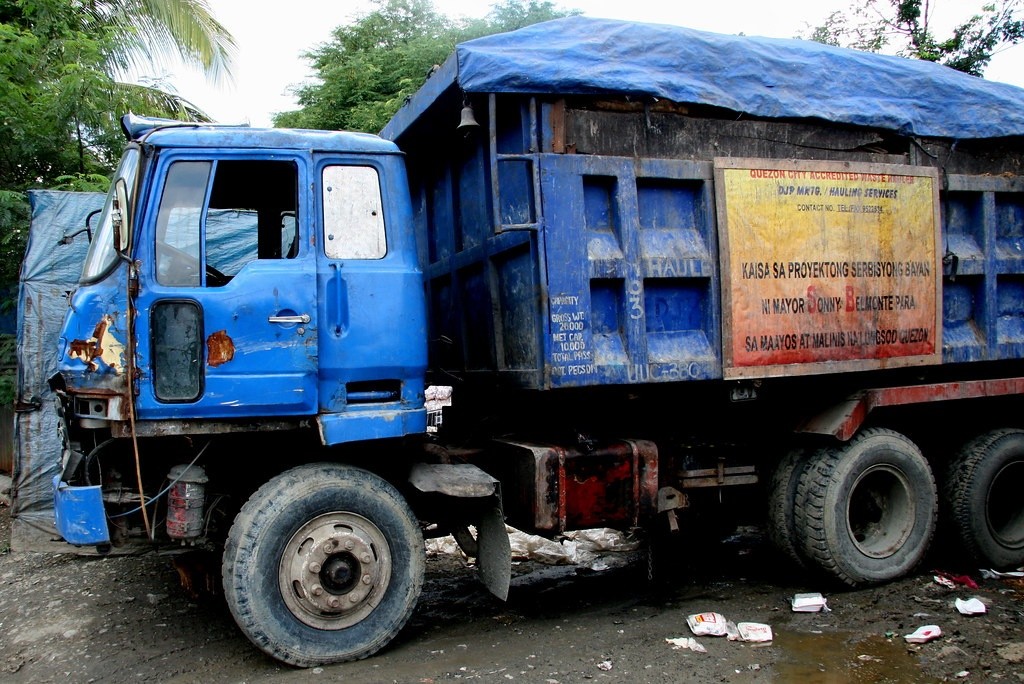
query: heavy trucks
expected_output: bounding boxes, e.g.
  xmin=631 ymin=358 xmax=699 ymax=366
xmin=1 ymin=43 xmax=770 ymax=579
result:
xmin=43 ymin=15 xmax=1023 ymax=669
